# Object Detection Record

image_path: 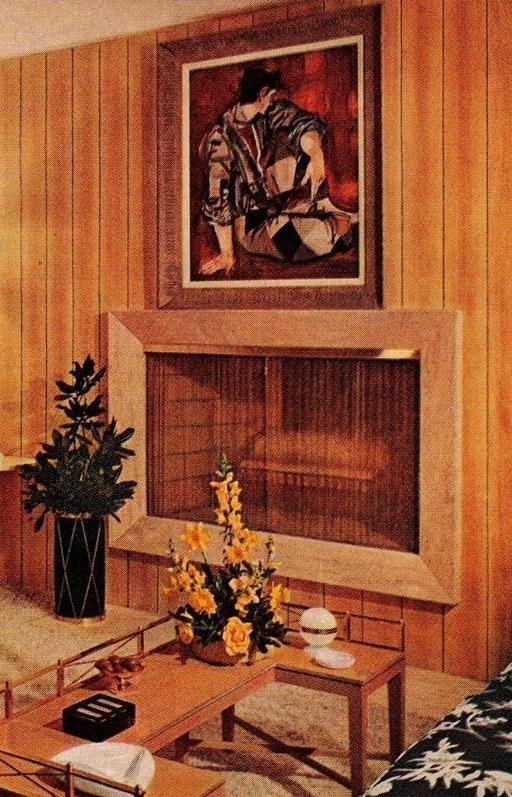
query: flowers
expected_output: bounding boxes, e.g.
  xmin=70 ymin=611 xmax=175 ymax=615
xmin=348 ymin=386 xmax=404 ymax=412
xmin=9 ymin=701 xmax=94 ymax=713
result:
xmin=161 ymin=450 xmax=300 ymax=658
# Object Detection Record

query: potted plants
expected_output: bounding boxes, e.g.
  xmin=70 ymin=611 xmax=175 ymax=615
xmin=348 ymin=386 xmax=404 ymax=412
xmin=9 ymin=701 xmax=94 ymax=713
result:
xmin=11 ymin=351 xmax=141 ymax=626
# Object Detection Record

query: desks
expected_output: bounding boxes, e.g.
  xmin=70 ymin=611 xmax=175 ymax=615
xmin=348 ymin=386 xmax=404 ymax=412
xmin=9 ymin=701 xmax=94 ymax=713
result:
xmin=0 ymin=602 xmax=406 ymax=795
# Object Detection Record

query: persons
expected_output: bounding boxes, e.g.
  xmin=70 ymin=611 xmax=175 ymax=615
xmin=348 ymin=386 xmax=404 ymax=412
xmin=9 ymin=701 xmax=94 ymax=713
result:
xmin=197 ymin=69 xmax=359 ymax=276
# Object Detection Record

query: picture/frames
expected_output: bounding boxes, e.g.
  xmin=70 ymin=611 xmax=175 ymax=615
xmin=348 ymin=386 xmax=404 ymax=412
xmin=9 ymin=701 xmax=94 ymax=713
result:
xmin=138 ymin=5 xmax=383 ymax=307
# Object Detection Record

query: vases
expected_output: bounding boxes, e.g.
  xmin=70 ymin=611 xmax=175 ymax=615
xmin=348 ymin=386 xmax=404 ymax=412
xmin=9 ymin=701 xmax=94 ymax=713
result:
xmin=189 ymin=635 xmax=251 ymax=666
